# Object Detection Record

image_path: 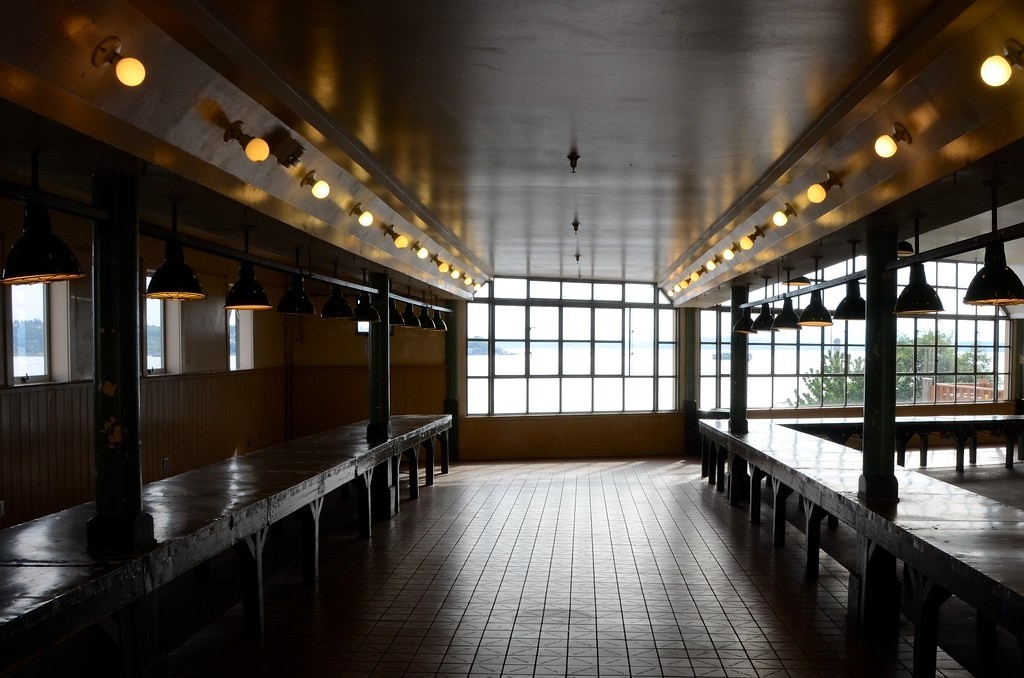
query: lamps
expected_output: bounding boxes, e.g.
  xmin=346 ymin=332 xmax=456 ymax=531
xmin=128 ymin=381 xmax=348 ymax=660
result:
xmin=91 ymin=193 xmax=450 ymax=333
xmin=730 ymin=154 xmax=1024 ymax=333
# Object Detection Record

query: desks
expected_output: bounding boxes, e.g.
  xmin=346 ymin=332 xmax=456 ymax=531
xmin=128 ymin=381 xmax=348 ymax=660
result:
xmin=19 ymin=413 xmax=453 ymax=677
xmin=697 ymin=414 xmax=1024 ymax=677
